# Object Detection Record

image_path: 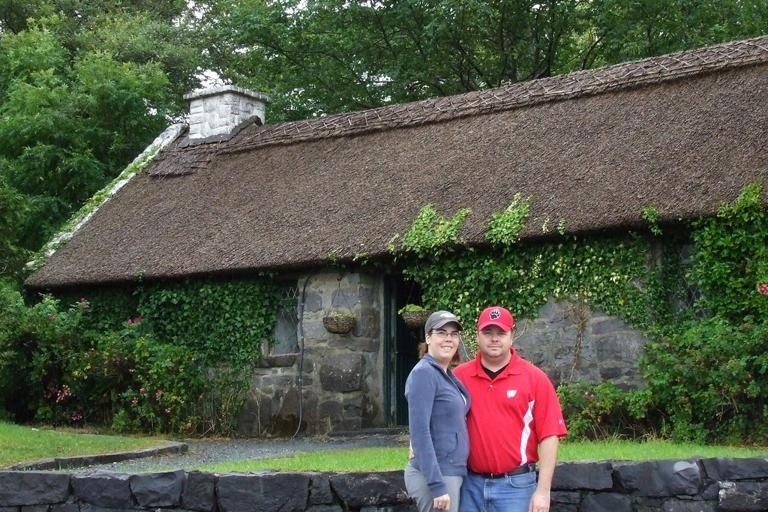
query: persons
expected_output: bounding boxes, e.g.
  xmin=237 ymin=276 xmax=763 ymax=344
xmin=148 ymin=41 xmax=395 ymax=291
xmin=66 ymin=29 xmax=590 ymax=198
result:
xmin=402 ymin=309 xmax=475 ymax=511
xmin=407 ymin=305 xmax=570 ymax=512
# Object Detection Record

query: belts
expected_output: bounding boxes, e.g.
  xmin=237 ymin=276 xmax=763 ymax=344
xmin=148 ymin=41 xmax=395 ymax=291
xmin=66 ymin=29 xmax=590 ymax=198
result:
xmin=467 ymin=463 xmax=535 ymax=479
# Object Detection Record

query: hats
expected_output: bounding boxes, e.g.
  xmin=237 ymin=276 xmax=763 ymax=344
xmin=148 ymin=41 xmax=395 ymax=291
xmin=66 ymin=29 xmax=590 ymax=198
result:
xmin=424 ymin=310 xmax=463 ymax=333
xmin=477 ymin=306 xmax=513 ymax=332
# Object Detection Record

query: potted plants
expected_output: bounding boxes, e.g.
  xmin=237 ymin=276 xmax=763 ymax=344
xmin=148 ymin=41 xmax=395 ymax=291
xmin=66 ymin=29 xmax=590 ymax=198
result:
xmin=322 ymin=309 xmax=356 ymax=336
xmin=397 ymin=303 xmax=434 ymax=330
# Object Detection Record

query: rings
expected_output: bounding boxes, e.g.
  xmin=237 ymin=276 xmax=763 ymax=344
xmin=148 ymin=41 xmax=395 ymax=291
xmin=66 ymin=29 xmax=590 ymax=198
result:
xmin=438 ymin=503 xmax=442 ymax=506
